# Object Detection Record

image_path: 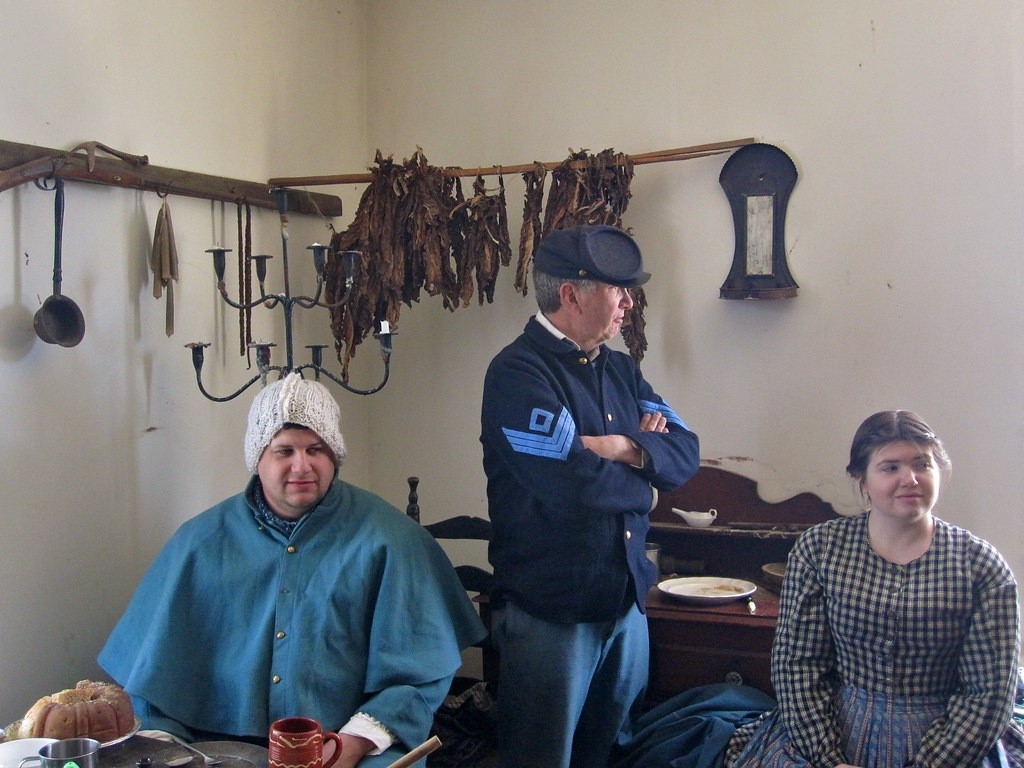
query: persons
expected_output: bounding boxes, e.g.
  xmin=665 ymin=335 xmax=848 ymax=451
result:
xmin=96 ymin=371 xmax=490 ymax=768
xmin=479 ymin=224 xmax=700 ymax=768
xmin=726 ymin=410 xmax=1024 ymax=768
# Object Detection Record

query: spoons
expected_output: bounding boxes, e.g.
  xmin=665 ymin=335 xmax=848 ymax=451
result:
xmin=172 ymin=734 xmax=222 ymax=765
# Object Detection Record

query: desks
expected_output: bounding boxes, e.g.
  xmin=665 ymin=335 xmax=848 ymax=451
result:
xmin=649 ymin=466 xmax=846 ymax=704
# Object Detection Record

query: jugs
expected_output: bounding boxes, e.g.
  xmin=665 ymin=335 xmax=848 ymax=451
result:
xmin=671 ymin=507 xmax=717 ymax=527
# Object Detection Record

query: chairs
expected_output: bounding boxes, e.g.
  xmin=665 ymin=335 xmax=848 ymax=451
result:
xmin=407 ymin=476 xmax=497 ymax=768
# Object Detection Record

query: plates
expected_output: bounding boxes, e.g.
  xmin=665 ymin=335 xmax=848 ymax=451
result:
xmin=99 ymin=714 xmax=142 ymax=749
xmin=656 ymin=576 xmax=758 ymax=605
xmin=0 ymin=738 xmax=60 ymax=768
xmin=132 ymin=740 xmax=269 ymax=768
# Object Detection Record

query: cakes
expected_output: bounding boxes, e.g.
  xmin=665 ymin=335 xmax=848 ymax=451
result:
xmin=18 ymin=679 xmax=134 ymax=746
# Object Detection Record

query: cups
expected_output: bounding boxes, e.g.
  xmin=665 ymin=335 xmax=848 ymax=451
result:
xmin=268 ymin=717 xmax=343 ymax=768
xmin=644 ymin=542 xmax=662 ymax=577
xmin=17 ymin=737 xmax=101 ymax=768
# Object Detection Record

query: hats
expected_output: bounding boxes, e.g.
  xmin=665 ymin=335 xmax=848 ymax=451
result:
xmin=245 ymin=372 xmax=346 ymax=475
xmin=534 ymin=225 xmax=652 ymax=287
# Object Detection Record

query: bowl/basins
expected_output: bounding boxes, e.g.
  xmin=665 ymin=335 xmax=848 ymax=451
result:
xmin=762 ymin=562 xmax=789 ymax=593
